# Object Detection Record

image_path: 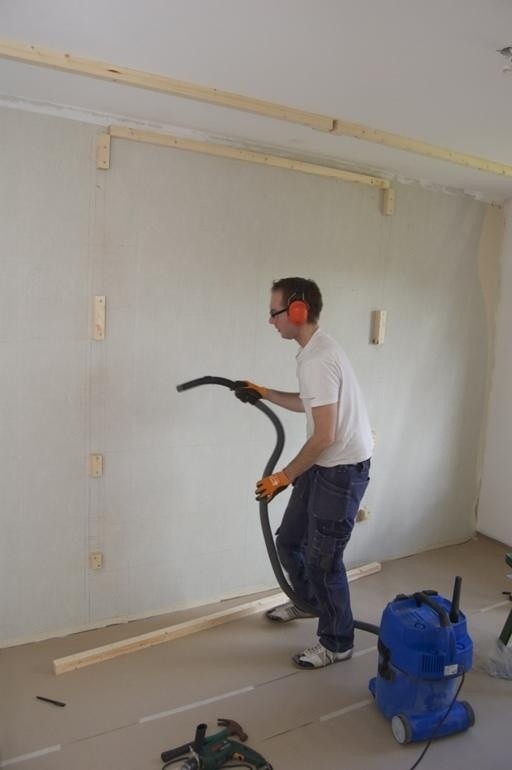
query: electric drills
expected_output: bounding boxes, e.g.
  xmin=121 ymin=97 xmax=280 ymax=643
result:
xmin=181 ymin=738 xmax=273 ymax=770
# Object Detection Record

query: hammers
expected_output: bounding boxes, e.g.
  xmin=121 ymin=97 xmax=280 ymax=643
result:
xmin=161 ymin=719 xmax=248 ymax=762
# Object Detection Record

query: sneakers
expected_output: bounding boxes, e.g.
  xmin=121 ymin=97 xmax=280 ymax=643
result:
xmin=291 ymin=643 xmax=352 ymax=670
xmin=265 ymin=601 xmax=318 ymax=622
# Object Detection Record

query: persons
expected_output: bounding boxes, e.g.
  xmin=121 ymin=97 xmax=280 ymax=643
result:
xmin=228 ymin=276 xmax=377 ymax=672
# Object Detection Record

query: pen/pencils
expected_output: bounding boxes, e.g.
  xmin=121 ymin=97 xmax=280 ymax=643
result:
xmin=36 ymin=697 xmax=65 ymax=707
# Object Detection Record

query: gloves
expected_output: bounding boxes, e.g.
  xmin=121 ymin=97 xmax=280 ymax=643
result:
xmin=255 ymin=471 xmax=291 ymax=503
xmin=230 ymin=380 xmax=268 ymax=404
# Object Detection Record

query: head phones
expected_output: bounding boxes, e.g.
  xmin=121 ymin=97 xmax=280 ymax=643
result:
xmin=286 ymin=277 xmax=310 ymax=325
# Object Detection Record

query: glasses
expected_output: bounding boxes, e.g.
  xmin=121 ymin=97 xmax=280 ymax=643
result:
xmin=270 ymin=309 xmax=286 ymax=319
